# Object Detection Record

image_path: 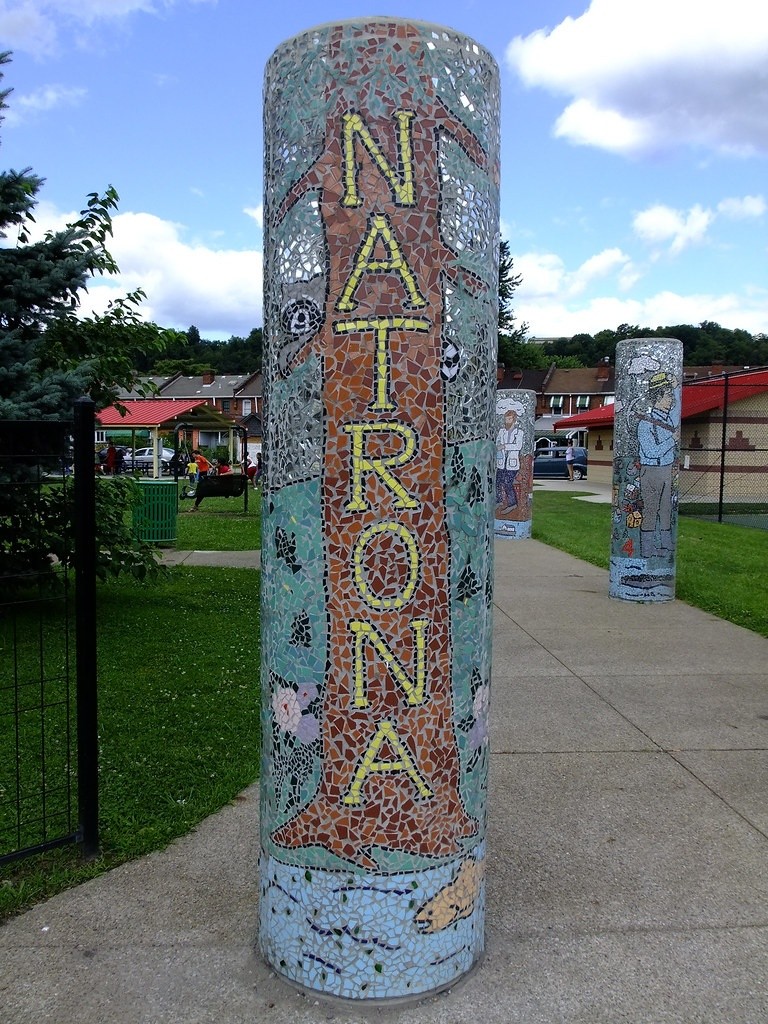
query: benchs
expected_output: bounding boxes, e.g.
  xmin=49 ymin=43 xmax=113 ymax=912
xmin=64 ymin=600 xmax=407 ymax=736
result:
xmin=178 ymin=474 xmax=249 ymax=512
xmin=122 ymin=465 xmax=175 ymax=470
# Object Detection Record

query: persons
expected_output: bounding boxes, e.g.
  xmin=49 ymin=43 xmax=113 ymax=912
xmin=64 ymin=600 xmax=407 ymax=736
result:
xmin=565 ymin=441 xmax=575 ymax=481
xmin=185 ymin=457 xmax=198 ymax=487
xmin=116 ymin=449 xmax=124 ymax=474
xmin=255 ymin=453 xmax=263 ymax=485
xmin=238 ymin=452 xmax=258 ymax=489
xmin=95 ymin=442 xmax=116 ymax=477
xmin=191 ymin=449 xmax=215 ymax=481
xmin=216 ymin=458 xmax=233 ymax=476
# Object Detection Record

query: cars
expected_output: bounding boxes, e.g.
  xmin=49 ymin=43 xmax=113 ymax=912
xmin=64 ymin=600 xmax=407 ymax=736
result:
xmin=122 ymin=446 xmax=180 ymax=475
xmin=534 ymin=443 xmax=588 ymax=480
xmin=97 ymin=445 xmax=137 ymax=471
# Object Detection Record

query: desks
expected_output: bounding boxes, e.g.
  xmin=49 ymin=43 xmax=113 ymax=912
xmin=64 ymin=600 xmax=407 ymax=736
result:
xmin=122 ymin=459 xmax=144 ymax=473
xmin=135 ymin=461 xmax=170 ymax=477
